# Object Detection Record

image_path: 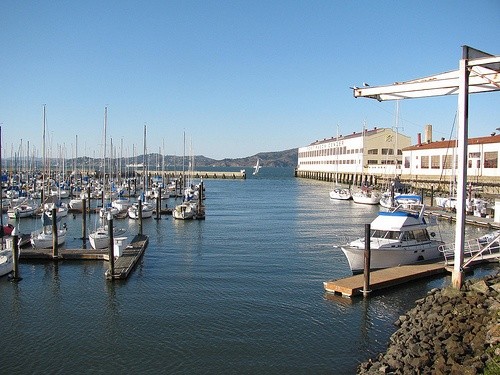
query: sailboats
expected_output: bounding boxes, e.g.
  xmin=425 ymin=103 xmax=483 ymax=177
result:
xmin=0 ymin=127 xmax=205 ymax=283
xmin=88 ymin=107 xmax=111 ymax=251
xmin=30 ymin=103 xmax=67 ymax=250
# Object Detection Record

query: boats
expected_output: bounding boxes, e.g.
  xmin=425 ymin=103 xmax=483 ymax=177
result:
xmin=379 ymin=191 xmax=421 ymax=207
xmin=332 ymin=204 xmax=445 ymax=276
xmin=329 ymin=188 xmax=350 ymax=199
xmin=350 ymin=186 xmax=381 ymax=203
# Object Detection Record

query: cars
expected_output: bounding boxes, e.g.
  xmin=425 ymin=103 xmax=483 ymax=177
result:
xmin=435 ymin=196 xmax=486 ymax=214
